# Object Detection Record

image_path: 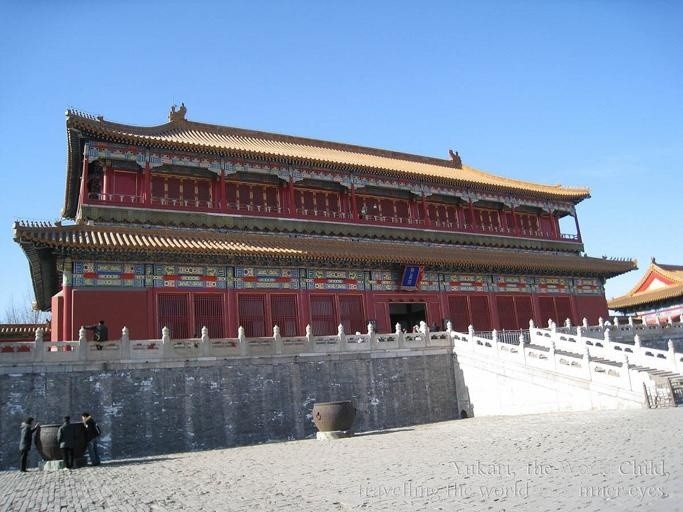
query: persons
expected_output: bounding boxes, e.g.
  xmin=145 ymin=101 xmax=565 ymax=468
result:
xmin=91 ymin=183 xmax=100 ymax=199
xmin=19 ymin=417 xmax=41 ymax=472
xmin=360 ymin=202 xmax=367 ymax=216
xmin=412 ymin=320 xmax=441 ymax=340
xmin=544 ymin=320 xmax=576 ymax=328
xmin=57 ymin=415 xmax=76 ymax=468
xmin=662 ymin=319 xmax=671 ymax=328
xmin=82 ymin=412 xmax=101 ymax=465
xmin=84 ymin=321 xmax=109 ymax=350
